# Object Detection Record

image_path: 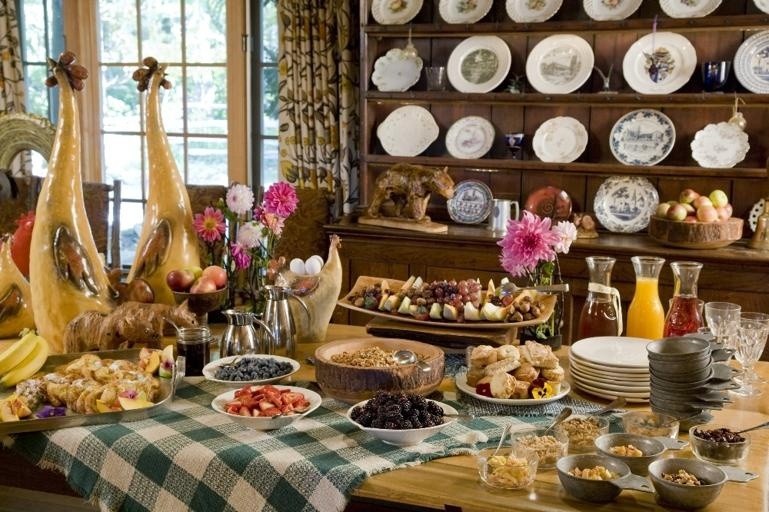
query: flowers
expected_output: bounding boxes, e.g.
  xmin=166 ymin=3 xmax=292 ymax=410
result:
xmin=193 ymin=181 xmax=300 ymax=328
xmin=497 ymin=209 xmax=578 ymax=349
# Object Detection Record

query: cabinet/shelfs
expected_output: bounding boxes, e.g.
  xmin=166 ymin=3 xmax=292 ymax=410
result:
xmin=323 ymin=0 xmax=769 ymax=362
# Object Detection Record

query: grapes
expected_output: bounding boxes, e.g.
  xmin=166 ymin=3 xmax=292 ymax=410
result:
xmin=406 ymin=278 xmax=481 ymax=309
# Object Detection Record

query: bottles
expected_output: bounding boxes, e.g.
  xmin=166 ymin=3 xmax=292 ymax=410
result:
xmin=576 ymin=256 xmax=704 ymax=339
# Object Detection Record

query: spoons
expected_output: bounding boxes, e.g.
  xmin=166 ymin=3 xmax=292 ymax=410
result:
xmin=392 ymin=350 xmax=432 ymax=373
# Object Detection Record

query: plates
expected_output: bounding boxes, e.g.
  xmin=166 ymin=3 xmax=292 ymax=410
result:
xmin=504 ymin=2 xmax=564 ymax=25
xmin=524 ymin=185 xmax=572 ymax=227
xmin=445 ymin=115 xmax=494 ymax=161
xmin=436 ymin=0 xmax=494 ymax=25
xmin=372 ymin=47 xmax=425 ymax=94
xmin=455 ymin=372 xmax=571 ymax=405
xmin=622 ymin=32 xmax=698 ymax=95
xmin=377 ymin=106 xmax=441 ymax=160
xmin=531 ymin=114 xmax=591 ymax=163
xmin=568 ymin=336 xmax=655 ymax=403
xmin=594 ymin=175 xmax=661 ymax=238
xmin=446 ymin=35 xmax=512 ymax=97
xmin=691 ymin=121 xmax=751 ymax=172
xmin=609 ymin=109 xmax=678 ymax=168
xmin=749 ymin=200 xmax=768 ymax=233
xmin=585 ymin=0 xmax=641 ymax=19
xmin=733 ymin=30 xmax=769 ymax=97
xmin=659 ymin=1 xmax=723 ymax=20
xmin=445 ymin=179 xmax=490 ymax=226
xmin=371 ymin=1 xmax=424 ymax=24
xmin=521 ymin=35 xmax=595 ymax=98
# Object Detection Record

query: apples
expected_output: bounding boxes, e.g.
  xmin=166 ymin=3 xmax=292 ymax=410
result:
xmin=658 ymin=188 xmax=733 ymax=223
xmin=160 ymin=263 xmax=230 ymax=294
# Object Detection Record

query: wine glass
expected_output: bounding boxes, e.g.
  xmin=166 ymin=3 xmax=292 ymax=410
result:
xmin=705 ymin=302 xmax=769 ymax=398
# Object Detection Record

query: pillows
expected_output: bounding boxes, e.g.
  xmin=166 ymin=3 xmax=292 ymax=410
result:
xmin=0 ymin=323 xmax=769 ymax=512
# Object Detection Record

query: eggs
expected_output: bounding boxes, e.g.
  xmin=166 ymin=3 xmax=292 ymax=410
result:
xmin=289 ymin=254 xmax=324 ymax=276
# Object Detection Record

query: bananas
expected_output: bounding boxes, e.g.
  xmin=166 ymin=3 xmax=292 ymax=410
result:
xmin=0 ymin=327 xmax=48 ymax=387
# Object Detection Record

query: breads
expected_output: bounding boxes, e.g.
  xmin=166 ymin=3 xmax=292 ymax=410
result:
xmin=461 ymin=341 xmax=564 ymax=399
xmin=42 ymin=349 xmax=166 ymax=416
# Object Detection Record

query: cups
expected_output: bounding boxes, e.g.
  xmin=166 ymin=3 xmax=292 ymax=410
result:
xmin=489 ymin=193 xmax=519 ymax=233
xmin=425 ymin=65 xmax=448 ymax=92
xmin=178 ymin=327 xmax=212 ymax=375
xmin=699 ymin=60 xmax=730 ymax=92
xmin=503 ymin=129 xmax=528 ymax=159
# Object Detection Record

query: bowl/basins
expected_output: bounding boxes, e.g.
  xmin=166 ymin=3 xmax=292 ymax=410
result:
xmin=646 ymin=333 xmax=744 ymax=431
xmin=201 ymin=354 xmax=460 ymax=446
xmin=555 ymin=432 xmax=761 ymax=508
xmin=477 ymin=410 xmax=751 ymax=490
